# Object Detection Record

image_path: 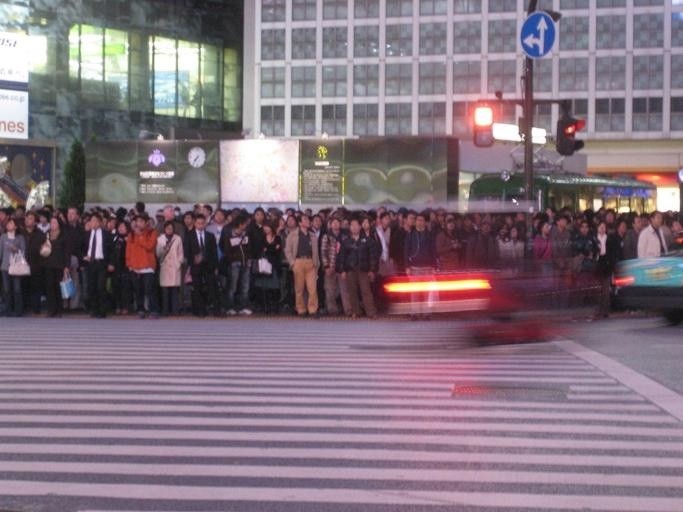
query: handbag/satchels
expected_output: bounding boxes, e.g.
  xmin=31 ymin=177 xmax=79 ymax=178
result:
xmin=59 ymin=277 xmax=76 ymax=300
xmin=257 ymin=258 xmax=273 ymax=276
xmin=8 ymin=262 xmax=31 ymax=276
xmin=41 ymin=240 xmax=52 ymax=258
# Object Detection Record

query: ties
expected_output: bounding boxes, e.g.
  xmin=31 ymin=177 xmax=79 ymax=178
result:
xmin=90 ymin=231 xmax=96 ymax=261
xmin=200 ymin=233 xmax=203 ymax=247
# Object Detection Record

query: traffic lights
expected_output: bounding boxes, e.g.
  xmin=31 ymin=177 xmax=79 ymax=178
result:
xmin=557 ymin=114 xmax=586 ymax=155
xmin=472 ymin=99 xmax=494 ymax=146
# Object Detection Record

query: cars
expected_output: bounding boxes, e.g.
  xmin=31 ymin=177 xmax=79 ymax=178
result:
xmin=608 ymin=246 xmax=683 ymax=325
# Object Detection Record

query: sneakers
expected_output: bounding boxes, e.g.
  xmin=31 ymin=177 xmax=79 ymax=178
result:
xmin=89 ymin=309 xmax=158 ymax=319
xmin=196 ymin=308 xmax=252 ymax=317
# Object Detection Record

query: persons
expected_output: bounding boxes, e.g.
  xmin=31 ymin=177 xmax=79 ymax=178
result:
xmin=214 ymin=207 xmax=450 ymax=319
xmin=1 ymin=202 xmax=227 ymax=317
xmin=437 ymin=205 xmax=683 ymax=318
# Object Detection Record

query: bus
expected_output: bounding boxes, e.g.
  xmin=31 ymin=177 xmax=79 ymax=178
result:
xmin=467 ymin=170 xmax=658 ymax=216
